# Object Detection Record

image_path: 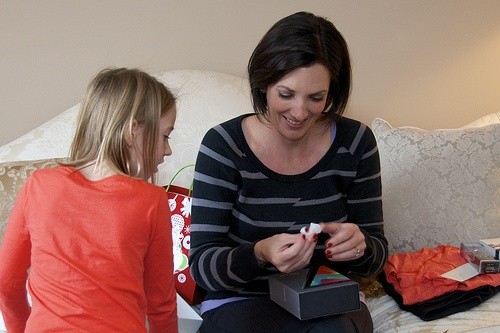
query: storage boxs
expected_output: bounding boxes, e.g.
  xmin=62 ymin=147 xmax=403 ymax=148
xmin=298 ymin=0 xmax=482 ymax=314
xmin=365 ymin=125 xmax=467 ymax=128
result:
xmin=269 ymin=265 xmax=362 ymax=320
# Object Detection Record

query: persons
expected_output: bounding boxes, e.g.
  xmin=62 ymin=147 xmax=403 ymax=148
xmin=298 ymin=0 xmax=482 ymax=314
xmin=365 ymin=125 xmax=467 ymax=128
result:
xmin=189 ymin=11 xmax=388 ymax=333
xmin=0 ymin=68 xmax=179 ymax=333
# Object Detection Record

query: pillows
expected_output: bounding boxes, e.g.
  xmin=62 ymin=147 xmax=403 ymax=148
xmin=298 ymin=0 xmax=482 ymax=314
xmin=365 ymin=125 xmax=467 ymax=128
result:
xmin=372 ymin=117 xmax=500 ymax=255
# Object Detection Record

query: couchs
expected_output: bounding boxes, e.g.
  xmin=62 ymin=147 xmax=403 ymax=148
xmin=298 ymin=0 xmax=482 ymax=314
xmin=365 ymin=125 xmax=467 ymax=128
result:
xmin=0 ymin=70 xmax=500 ymax=333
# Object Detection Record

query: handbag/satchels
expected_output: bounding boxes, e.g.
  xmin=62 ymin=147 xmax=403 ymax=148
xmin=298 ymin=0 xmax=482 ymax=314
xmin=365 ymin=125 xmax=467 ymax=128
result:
xmin=160 ymin=164 xmax=206 ymax=306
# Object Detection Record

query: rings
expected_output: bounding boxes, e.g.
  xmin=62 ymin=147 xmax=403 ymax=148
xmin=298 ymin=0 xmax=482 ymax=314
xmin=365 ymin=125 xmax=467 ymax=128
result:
xmin=354 ymin=246 xmax=360 ymax=258
